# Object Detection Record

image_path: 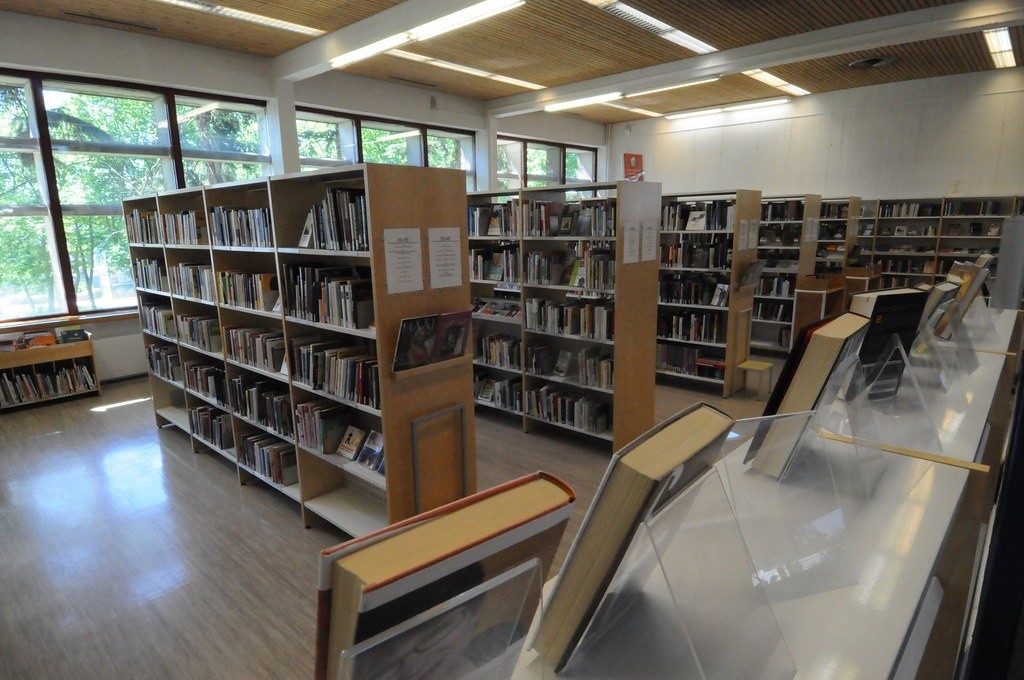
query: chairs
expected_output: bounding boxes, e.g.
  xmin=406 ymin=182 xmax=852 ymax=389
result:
xmin=733 ymin=306 xmax=775 ymax=400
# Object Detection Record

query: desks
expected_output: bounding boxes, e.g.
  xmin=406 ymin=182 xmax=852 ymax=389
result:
xmin=844 ymin=274 xmax=883 ymax=305
xmin=790 ymin=288 xmax=846 ymax=350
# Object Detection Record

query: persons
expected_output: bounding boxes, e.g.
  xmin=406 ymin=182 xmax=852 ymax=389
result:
xmin=407 ymin=327 xmax=429 ymax=365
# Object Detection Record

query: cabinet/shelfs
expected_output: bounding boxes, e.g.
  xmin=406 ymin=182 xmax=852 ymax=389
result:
xmin=463 ymin=181 xmax=664 ymax=455
xmin=0 ymin=330 xmax=102 ymax=410
xmin=683 ymin=193 xmax=823 ymax=354
xmin=565 ymin=189 xmax=763 ymax=398
xmin=123 ymin=162 xmax=480 ymax=539
xmin=762 ymin=196 xmax=863 ymax=277
xmin=824 ymin=195 xmax=1024 ymax=301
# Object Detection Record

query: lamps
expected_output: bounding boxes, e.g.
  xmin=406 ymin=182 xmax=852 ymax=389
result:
xmin=329 ymin=0 xmax=527 ymax=86
xmin=544 ymin=53 xmax=721 ymax=113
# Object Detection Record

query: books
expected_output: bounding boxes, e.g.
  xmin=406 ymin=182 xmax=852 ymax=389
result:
xmin=743 ymin=309 xmax=871 ymax=485
xmin=313 ymin=471 xmax=574 ymax=679
xmin=467 ymin=199 xmax=615 ymax=433
xmin=914 ymin=254 xmax=996 ymax=343
xmin=853 ymin=202 xmax=1000 ymax=286
xmin=0 ymin=327 xmax=100 ymax=407
xmin=835 ymin=288 xmax=928 ymax=404
xmin=655 ymin=200 xmax=735 ymax=378
xmin=526 ymin=403 xmax=734 ymax=670
xmin=392 ymin=311 xmax=471 ymax=372
xmin=740 ymin=199 xmax=802 ymax=344
xmin=815 ymin=201 xmax=849 ymax=277
xmin=121 ymin=187 xmax=387 ymax=486
